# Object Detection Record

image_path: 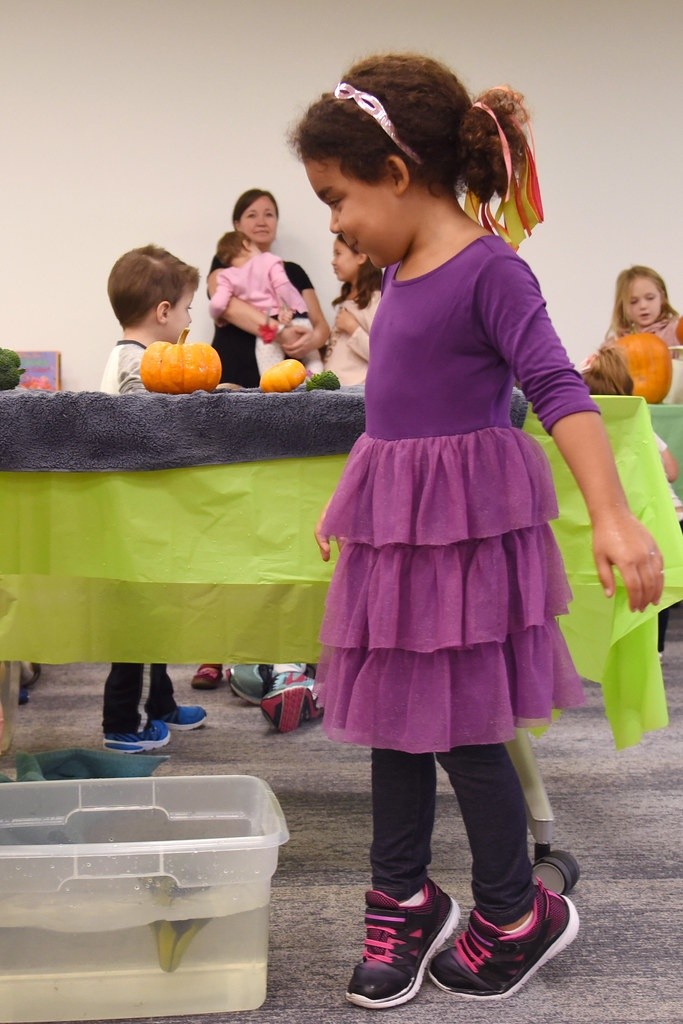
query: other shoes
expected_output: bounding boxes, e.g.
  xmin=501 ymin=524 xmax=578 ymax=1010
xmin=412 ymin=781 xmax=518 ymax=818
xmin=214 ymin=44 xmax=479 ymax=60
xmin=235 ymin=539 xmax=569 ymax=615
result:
xmin=191 ymin=663 xmax=223 ymax=690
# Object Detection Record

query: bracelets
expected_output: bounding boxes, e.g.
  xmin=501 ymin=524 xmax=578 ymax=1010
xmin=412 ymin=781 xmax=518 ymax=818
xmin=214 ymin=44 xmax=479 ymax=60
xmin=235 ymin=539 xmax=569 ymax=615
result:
xmin=275 ymin=324 xmax=286 ymax=334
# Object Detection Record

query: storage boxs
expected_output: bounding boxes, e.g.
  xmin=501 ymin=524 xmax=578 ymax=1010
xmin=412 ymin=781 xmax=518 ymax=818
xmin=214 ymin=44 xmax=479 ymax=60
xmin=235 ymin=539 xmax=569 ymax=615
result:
xmin=0 ymin=774 xmax=290 ymax=1022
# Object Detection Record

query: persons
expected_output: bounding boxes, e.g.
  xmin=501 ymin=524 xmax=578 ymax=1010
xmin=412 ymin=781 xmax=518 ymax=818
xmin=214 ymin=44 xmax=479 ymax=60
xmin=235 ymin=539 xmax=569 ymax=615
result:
xmin=191 ymin=190 xmax=382 ymax=692
xmin=103 ymin=244 xmax=208 ymax=755
xmin=581 ymin=267 xmax=683 ymax=665
xmin=296 ymin=54 xmax=664 ymax=1010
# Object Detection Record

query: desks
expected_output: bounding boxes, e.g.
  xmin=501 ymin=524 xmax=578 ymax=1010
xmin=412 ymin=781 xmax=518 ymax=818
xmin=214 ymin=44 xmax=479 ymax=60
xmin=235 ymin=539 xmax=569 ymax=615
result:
xmin=0 ymin=386 xmax=581 ymax=895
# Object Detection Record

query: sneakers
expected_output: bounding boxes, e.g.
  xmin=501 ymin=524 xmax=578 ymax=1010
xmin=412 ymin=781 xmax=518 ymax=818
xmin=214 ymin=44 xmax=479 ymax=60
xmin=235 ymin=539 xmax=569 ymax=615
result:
xmin=226 ymin=662 xmax=317 ymax=706
xmin=428 ymin=875 xmax=580 ymax=1001
xmin=259 ymin=670 xmax=326 ymax=732
xmin=167 ymin=706 xmax=207 ymax=731
xmin=103 ymin=719 xmax=171 ymax=754
xmin=346 ymin=878 xmax=461 ymax=1009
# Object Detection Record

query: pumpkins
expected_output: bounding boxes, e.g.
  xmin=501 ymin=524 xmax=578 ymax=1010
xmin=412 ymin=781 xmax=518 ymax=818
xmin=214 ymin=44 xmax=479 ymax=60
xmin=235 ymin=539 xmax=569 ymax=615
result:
xmin=613 ymin=323 xmax=673 ymax=406
xmin=260 ymin=359 xmax=307 ymax=392
xmin=140 ymin=329 xmax=222 ymax=394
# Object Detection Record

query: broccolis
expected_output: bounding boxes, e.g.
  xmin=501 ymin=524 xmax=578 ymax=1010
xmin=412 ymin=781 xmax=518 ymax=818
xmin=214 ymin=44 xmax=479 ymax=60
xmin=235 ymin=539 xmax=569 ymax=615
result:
xmin=0 ymin=348 xmax=26 ymax=391
xmin=306 ymin=369 xmax=341 ymax=391
